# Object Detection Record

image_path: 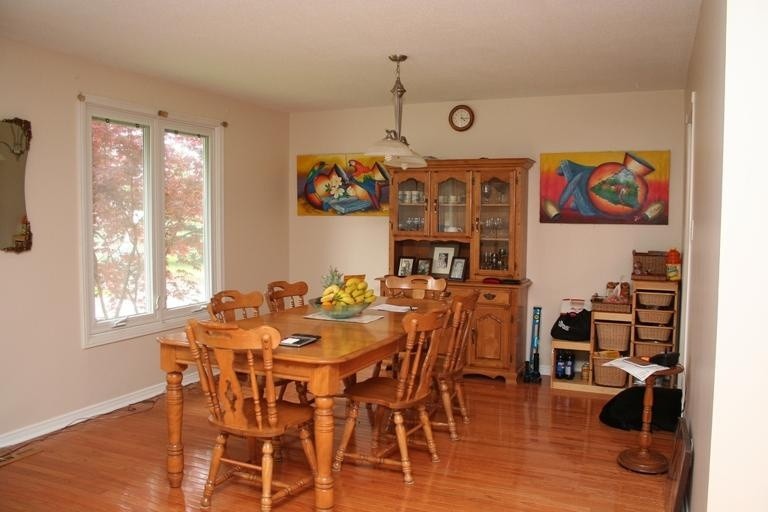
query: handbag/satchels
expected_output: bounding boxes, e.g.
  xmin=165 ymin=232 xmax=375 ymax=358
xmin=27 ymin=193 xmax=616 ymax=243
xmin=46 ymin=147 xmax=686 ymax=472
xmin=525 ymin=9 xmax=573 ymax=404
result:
xmin=551 ymin=309 xmax=591 ymax=341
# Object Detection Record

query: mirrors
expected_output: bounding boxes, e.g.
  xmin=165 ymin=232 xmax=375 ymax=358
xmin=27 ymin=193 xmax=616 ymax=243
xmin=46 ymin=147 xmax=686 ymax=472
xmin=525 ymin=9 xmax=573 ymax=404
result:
xmin=1 ymin=117 xmax=33 ymax=255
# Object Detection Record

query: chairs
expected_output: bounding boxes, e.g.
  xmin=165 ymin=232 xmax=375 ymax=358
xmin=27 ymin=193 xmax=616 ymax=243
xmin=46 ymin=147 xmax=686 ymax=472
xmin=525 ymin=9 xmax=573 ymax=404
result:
xmin=330 ymin=302 xmax=452 ymax=484
xmin=262 ymin=279 xmax=357 ymax=406
xmin=187 ymin=320 xmax=316 ymax=511
xmin=364 ymin=272 xmax=448 ymax=410
xmin=382 ymin=288 xmax=481 ymax=442
xmin=206 ymin=289 xmax=308 ymax=405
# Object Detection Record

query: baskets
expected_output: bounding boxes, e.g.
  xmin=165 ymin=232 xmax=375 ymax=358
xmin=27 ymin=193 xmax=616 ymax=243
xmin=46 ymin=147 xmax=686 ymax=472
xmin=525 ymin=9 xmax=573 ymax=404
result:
xmin=594 ymin=322 xmax=631 ymax=351
xmin=634 ymin=341 xmax=673 ymax=358
xmin=636 ymin=309 xmax=674 ymax=324
xmin=635 ymin=325 xmax=673 ymax=341
xmin=633 ymin=250 xmax=667 ymax=276
xmin=591 ymin=303 xmax=632 ymax=314
xmin=636 ymin=292 xmax=675 ymax=307
xmin=592 ymin=357 xmax=627 ymax=387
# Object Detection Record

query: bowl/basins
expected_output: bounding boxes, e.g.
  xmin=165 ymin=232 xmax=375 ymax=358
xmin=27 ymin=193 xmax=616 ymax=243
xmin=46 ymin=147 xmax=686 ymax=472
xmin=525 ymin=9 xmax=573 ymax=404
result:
xmin=308 ymin=298 xmax=372 ymax=319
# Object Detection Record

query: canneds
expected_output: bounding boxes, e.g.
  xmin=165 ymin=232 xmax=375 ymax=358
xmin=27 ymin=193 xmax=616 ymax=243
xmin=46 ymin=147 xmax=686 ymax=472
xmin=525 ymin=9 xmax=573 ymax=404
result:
xmin=582 ymin=363 xmax=589 ymax=380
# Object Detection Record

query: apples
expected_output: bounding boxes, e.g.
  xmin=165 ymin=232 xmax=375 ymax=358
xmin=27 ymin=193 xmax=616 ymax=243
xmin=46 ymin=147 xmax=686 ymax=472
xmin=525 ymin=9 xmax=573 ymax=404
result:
xmin=634 ymin=262 xmax=649 ymax=276
xmin=608 ymin=281 xmax=629 ymax=304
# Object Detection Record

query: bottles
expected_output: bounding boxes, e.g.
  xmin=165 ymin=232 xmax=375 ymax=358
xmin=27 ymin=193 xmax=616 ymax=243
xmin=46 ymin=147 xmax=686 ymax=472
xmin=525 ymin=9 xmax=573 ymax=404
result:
xmin=556 ymin=350 xmax=575 ymax=379
xmin=665 ymin=250 xmax=682 ymax=282
xmin=405 ymin=216 xmax=424 ymax=231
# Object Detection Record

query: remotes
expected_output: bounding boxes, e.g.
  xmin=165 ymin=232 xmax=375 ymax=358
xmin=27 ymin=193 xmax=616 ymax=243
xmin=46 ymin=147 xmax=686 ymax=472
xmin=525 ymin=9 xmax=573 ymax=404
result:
xmin=292 ymin=334 xmax=321 ymax=339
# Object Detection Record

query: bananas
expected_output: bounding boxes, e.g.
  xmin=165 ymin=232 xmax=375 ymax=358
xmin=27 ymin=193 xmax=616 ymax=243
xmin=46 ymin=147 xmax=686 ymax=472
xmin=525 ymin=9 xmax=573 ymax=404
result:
xmin=320 ymin=285 xmax=354 ymax=305
xmin=345 ymin=278 xmax=377 ymax=304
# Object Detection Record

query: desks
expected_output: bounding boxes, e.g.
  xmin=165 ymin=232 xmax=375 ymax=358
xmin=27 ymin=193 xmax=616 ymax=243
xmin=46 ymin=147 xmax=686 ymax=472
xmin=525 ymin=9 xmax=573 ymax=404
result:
xmin=615 ymin=360 xmax=684 ymax=478
xmin=153 ymin=296 xmax=455 ymax=511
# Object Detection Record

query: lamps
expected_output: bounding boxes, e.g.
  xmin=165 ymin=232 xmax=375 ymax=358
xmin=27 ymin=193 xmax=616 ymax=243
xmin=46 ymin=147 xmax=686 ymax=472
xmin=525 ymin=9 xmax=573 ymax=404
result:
xmin=361 ymin=52 xmax=428 ymax=171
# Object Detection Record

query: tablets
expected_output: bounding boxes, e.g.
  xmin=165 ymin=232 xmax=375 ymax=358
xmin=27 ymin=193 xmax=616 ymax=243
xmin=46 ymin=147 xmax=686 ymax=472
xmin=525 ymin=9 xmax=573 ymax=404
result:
xmin=279 ymin=335 xmax=317 ymax=347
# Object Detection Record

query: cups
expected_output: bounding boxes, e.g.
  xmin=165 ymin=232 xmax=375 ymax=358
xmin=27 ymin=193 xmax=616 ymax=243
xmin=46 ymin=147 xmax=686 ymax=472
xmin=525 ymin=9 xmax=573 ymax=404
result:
xmin=438 ymin=195 xmax=459 ymax=204
xmin=481 ymin=216 xmax=508 ymax=238
xmin=481 ymin=183 xmax=506 ymax=204
xmin=398 ymin=190 xmax=425 ymax=204
xmin=480 ymin=248 xmax=508 ymax=271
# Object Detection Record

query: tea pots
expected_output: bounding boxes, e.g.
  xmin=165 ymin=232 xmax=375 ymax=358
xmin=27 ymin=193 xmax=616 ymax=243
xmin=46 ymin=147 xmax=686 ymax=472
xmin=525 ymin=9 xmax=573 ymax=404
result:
xmin=444 ymin=227 xmax=462 ymax=232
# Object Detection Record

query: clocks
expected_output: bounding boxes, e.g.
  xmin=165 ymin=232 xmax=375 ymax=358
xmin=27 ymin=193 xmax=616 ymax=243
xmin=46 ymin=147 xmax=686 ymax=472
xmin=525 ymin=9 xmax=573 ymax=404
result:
xmin=447 ymin=104 xmax=474 ymax=132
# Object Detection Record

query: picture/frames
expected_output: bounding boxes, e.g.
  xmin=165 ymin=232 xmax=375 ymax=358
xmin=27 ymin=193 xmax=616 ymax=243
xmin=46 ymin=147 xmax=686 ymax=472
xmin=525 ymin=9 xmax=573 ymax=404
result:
xmin=395 ymin=256 xmax=415 ymax=277
xmin=448 ymin=255 xmax=469 ymax=281
xmin=413 ymin=258 xmax=432 ymax=276
xmin=428 ymin=244 xmax=459 ymax=276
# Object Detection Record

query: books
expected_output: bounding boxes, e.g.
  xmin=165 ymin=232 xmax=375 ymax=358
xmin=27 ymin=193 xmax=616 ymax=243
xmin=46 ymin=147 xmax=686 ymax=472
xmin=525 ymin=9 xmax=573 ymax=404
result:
xmin=624 ymin=356 xmax=658 ymax=368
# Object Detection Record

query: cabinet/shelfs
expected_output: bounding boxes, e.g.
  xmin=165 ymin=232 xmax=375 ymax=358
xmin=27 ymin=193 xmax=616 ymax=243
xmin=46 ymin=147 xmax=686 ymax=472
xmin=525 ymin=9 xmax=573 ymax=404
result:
xmin=472 ymin=158 xmax=536 ymax=282
xmin=374 ymin=277 xmax=535 ymax=386
xmin=384 ymin=158 xmax=472 ymax=281
xmin=549 ymin=279 xmax=680 ymax=397
